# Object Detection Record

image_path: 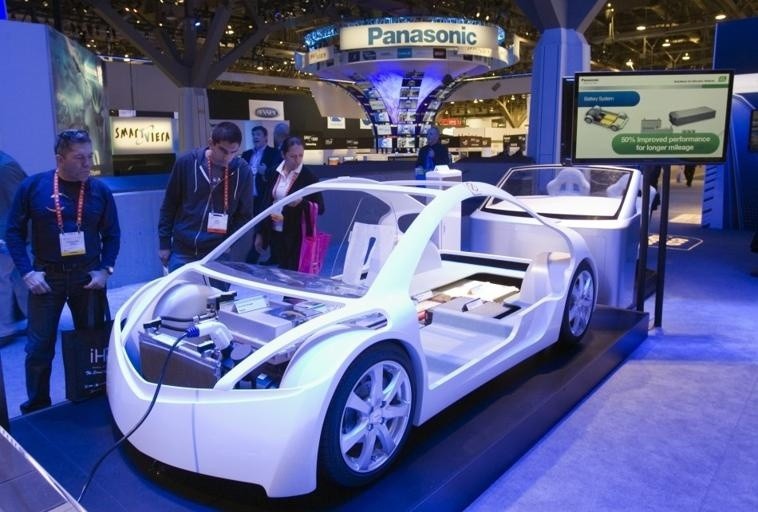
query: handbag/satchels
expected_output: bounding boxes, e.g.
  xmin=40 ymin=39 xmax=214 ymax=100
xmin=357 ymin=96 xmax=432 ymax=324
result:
xmin=60 ymin=275 xmax=127 ymax=405
xmin=297 ymin=198 xmax=332 ymax=275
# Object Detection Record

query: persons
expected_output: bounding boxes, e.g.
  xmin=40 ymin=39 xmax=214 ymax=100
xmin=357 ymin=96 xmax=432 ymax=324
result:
xmin=158 ymin=122 xmax=254 ymax=274
xmin=510 ymin=143 xmax=525 ymax=160
xmin=241 ymin=126 xmax=280 ymax=266
xmin=4 ymin=130 xmax=120 ymax=415
xmin=497 ymin=143 xmax=510 ymax=159
xmin=254 ymin=136 xmax=324 ymax=271
xmin=684 ymin=164 xmax=701 ymax=186
xmin=1 ymin=151 xmax=34 ymax=337
xmin=416 ymin=127 xmax=449 ymax=180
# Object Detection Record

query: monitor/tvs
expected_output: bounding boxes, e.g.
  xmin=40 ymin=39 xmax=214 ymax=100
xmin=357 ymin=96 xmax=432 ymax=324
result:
xmin=570 ymin=68 xmax=735 ymax=165
xmin=559 ymin=76 xmax=575 ymax=167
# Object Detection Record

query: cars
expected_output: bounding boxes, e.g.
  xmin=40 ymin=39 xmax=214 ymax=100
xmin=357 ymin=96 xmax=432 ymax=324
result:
xmin=103 ymin=174 xmax=601 ymax=500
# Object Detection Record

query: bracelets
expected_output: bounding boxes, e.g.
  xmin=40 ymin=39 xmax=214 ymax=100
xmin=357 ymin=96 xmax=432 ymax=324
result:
xmin=23 ymin=270 xmax=34 ymax=281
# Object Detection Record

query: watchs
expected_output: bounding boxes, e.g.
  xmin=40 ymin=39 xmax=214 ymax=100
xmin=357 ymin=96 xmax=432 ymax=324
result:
xmin=103 ymin=265 xmax=114 ymax=274
